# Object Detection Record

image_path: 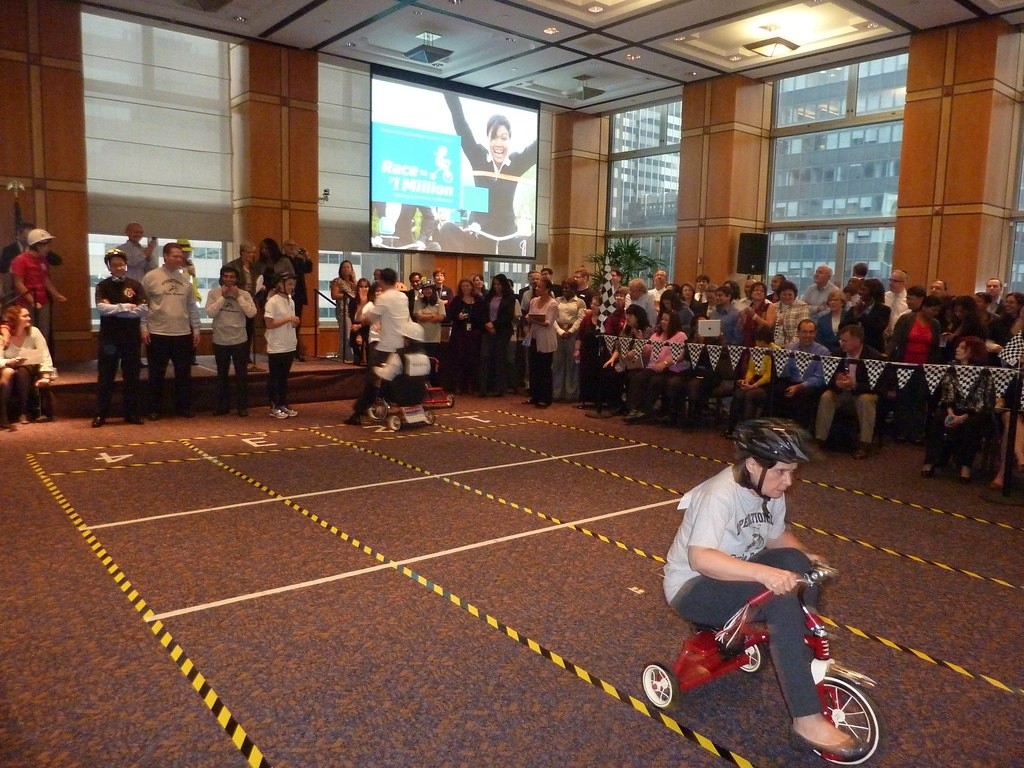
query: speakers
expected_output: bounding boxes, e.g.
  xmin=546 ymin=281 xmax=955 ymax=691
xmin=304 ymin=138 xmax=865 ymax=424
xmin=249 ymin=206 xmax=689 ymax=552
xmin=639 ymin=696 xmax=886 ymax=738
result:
xmin=736 ymin=233 xmax=768 ymax=275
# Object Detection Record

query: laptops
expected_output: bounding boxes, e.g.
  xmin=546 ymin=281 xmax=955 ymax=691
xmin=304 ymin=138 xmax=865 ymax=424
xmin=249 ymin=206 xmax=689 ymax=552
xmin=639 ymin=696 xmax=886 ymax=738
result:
xmin=698 ymin=319 xmax=721 ymax=337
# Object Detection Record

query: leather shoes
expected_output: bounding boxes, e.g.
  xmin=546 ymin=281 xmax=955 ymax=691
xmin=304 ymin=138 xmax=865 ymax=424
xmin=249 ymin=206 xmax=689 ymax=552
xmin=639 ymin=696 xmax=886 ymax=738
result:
xmin=125 ymin=413 xmax=145 ymax=425
xmin=149 ymin=413 xmax=159 ymax=421
xmin=176 ymin=407 xmax=196 ymax=418
xmin=92 ymin=415 xmax=106 ymax=427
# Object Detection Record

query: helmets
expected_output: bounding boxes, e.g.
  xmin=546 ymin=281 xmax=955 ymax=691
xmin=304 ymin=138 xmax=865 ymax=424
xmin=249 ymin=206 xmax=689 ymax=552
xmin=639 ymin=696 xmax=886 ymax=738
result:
xmin=104 ymin=247 xmax=130 ymax=271
xmin=732 ymin=417 xmax=810 ymax=470
xmin=26 ymin=228 xmax=56 ymax=248
xmin=219 ymin=262 xmax=242 ymax=288
xmin=398 ymin=322 xmax=425 ymax=342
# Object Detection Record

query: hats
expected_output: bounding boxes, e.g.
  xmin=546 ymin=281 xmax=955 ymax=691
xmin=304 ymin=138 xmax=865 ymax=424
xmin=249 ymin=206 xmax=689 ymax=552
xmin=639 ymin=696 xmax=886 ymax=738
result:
xmin=273 ymin=257 xmax=297 ymax=296
xmin=853 ymin=262 xmax=869 ymax=275
xmin=423 ymin=279 xmax=436 ymax=288
xmin=177 ymin=238 xmax=197 ymax=251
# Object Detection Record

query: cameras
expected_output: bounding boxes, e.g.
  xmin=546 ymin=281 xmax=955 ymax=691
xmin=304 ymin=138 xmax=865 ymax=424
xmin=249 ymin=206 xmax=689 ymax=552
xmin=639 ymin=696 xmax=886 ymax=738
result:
xmin=298 ymin=249 xmax=304 ymax=254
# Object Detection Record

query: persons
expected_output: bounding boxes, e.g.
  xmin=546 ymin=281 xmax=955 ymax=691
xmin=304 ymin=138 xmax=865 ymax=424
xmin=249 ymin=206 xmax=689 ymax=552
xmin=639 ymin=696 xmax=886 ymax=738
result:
xmin=0 ymin=305 xmax=57 ymax=427
xmin=663 ymin=417 xmax=870 ymax=758
xmin=219 ymin=237 xmax=313 ymax=364
xmin=9 ymin=228 xmax=68 ymax=342
xmin=92 ymin=248 xmax=148 ymax=428
xmin=372 ymin=89 xmax=537 ymax=258
xmin=205 ymin=264 xmax=257 ymax=417
xmin=115 ymin=222 xmax=156 ymax=366
xmin=263 ymin=270 xmax=300 ymax=419
xmin=0 ymin=223 xmax=62 ymax=365
xmin=177 ymin=239 xmax=202 ymax=365
xmin=140 ymin=243 xmax=200 ymax=419
xmin=330 ymin=259 xmax=1024 ymax=491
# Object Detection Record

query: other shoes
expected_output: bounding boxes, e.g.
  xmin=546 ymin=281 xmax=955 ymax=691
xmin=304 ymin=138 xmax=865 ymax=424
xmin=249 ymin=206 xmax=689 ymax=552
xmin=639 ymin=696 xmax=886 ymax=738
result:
xmin=961 ymin=474 xmax=971 ymax=485
xmin=788 ymin=727 xmax=871 ymax=760
xmin=920 ymin=465 xmax=935 ymax=477
xmin=989 ymin=472 xmax=1004 ymax=491
xmin=811 ymin=438 xmax=826 ymax=454
xmin=344 ymin=414 xmax=361 ymax=426
xmin=856 ymin=446 xmax=869 ymax=459
xmin=214 ymin=408 xmax=231 ymax=416
xmin=238 ymin=406 xmax=248 ymax=418
xmin=453 ymin=385 xmax=741 ymax=441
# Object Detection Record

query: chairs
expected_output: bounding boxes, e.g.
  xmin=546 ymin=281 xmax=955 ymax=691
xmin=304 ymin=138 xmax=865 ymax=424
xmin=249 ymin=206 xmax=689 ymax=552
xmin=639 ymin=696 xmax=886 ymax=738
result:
xmin=577 ymin=376 xmax=1000 ymax=479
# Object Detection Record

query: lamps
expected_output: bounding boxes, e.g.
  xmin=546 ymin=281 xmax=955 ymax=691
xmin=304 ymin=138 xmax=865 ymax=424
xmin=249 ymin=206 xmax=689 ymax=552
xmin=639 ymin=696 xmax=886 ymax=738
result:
xmin=402 ymin=32 xmax=454 ymax=63
xmin=561 ymin=74 xmax=606 ymax=101
xmin=743 ymin=25 xmax=800 ymax=58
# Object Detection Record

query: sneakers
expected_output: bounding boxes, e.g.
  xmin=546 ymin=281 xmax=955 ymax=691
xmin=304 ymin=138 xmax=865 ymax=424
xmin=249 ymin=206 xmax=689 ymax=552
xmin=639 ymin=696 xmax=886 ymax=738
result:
xmin=269 ymin=407 xmax=289 ymax=419
xmin=283 ymin=406 xmax=300 ymax=417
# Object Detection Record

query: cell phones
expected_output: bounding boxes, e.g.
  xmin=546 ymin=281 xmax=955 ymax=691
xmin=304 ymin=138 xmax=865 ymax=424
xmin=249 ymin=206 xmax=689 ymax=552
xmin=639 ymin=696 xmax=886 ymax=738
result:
xmin=153 ymin=236 xmax=157 ymax=240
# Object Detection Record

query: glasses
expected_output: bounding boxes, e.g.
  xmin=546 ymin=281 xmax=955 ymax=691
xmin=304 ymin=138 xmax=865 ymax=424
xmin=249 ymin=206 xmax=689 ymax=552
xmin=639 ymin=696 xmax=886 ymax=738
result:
xmin=890 ymin=277 xmax=904 ymax=283
xmin=838 ymin=338 xmax=852 ymax=343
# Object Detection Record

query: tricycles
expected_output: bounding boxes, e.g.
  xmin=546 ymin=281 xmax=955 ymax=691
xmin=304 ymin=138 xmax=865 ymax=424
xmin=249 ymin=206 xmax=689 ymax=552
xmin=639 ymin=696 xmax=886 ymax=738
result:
xmin=641 ymin=561 xmax=883 ymax=766
xmin=368 ymin=354 xmax=455 ymax=432
